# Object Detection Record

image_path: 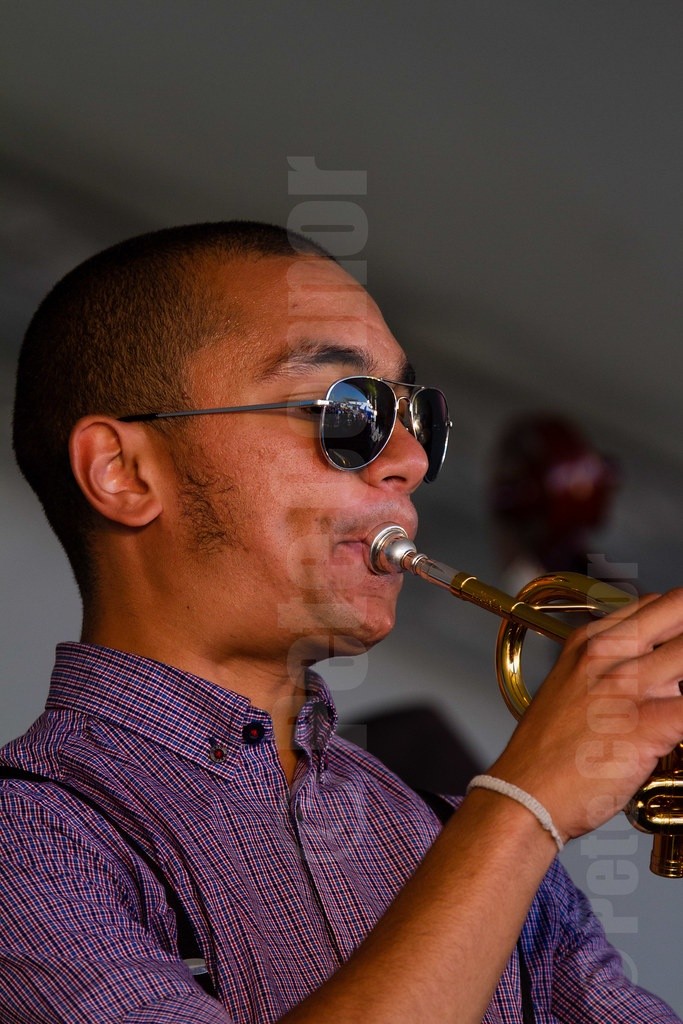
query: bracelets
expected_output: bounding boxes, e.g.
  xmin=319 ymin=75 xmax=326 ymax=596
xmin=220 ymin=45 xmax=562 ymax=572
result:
xmin=468 ymin=774 xmax=563 ymax=853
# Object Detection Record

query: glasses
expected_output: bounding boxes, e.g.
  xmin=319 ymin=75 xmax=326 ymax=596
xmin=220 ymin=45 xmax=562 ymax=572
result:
xmin=116 ymin=374 xmax=452 ymax=485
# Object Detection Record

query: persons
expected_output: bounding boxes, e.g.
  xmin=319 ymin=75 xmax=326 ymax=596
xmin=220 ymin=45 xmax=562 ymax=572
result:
xmin=1 ymin=221 xmax=682 ymax=1024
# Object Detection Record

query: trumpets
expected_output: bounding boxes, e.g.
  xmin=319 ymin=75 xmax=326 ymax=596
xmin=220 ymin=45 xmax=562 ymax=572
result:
xmin=362 ymin=522 xmax=682 ymax=884
